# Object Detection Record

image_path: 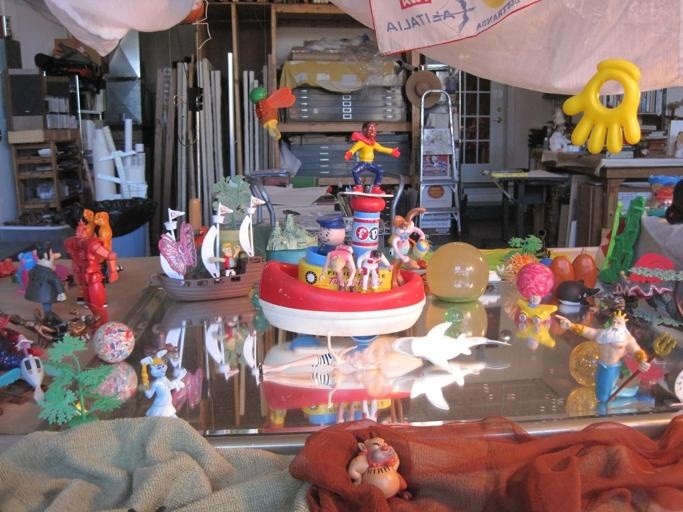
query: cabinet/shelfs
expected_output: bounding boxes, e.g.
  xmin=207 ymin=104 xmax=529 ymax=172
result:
xmin=270 ymin=4 xmax=421 ymax=229
xmin=10 ymin=137 xmax=85 ymax=213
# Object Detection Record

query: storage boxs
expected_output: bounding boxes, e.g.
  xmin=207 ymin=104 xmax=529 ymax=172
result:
xmin=9 ymin=75 xmax=78 ymax=130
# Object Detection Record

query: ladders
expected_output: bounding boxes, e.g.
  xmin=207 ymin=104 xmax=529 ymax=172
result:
xmin=416 ymin=89 xmax=462 ymax=236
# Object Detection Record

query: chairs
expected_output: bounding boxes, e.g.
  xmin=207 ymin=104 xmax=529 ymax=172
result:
xmin=482 ymin=147 xmax=682 ymax=248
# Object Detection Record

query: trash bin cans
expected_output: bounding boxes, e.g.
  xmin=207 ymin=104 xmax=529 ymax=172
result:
xmin=73 ymin=200 xmax=156 ymax=257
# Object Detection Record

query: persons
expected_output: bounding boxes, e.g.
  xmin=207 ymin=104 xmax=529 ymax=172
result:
xmin=344 ymin=121 xmax=401 ymax=194
xmin=555 ymin=312 xmax=651 ymax=373
xmin=316 ymin=214 xmax=393 ymax=294
xmin=209 ymin=241 xmax=241 ymax=277
xmin=218 ymin=322 xmax=243 ymax=351
xmin=0 ymin=60 xmax=682 ymax=502
xmin=263 ymin=373 xmax=348 ymax=408
xmin=26 ymin=210 xmax=119 ymax=333
xmin=259 ymin=321 xmax=398 ymax=373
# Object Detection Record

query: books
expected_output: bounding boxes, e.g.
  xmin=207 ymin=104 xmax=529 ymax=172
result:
xmin=43 ymin=97 xmax=78 ymax=128
xmin=604 ymin=125 xmax=669 ymax=158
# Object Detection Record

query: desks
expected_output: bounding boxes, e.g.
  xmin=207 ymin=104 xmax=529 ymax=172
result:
xmin=0 ymin=247 xmax=681 ymax=511
